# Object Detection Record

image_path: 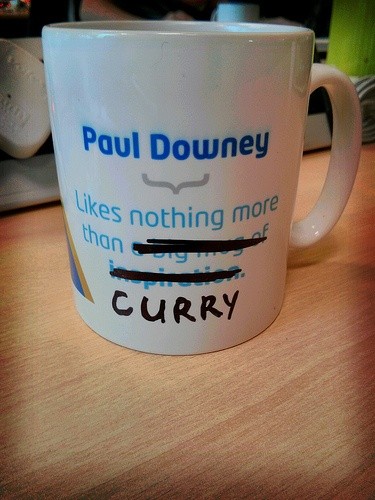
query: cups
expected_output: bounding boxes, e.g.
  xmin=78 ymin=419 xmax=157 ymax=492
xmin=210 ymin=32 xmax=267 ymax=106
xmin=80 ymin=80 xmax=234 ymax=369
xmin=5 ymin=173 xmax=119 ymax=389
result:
xmin=40 ymin=19 xmax=367 ymax=356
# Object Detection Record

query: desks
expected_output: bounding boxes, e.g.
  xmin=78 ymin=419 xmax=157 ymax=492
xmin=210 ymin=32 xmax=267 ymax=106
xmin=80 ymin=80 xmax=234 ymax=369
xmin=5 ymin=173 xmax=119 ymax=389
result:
xmin=2 ymin=139 xmax=374 ymax=499
